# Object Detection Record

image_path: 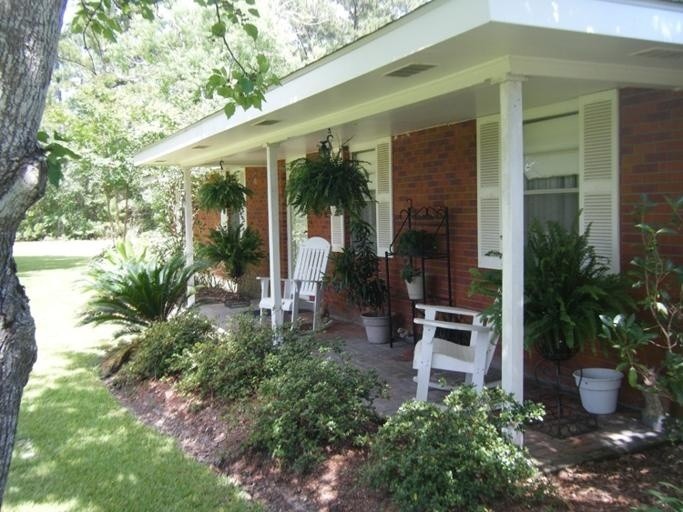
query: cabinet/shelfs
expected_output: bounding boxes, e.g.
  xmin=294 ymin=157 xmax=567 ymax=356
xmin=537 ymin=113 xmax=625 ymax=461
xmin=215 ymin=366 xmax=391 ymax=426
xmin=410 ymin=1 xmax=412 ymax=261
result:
xmin=383 ymin=199 xmax=457 ymax=348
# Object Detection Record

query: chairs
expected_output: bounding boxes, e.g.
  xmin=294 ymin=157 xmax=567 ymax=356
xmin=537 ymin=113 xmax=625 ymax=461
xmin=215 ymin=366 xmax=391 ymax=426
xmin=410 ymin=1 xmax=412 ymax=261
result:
xmin=411 ymin=288 xmax=503 ymax=414
xmin=254 ymin=235 xmax=334 ymax=336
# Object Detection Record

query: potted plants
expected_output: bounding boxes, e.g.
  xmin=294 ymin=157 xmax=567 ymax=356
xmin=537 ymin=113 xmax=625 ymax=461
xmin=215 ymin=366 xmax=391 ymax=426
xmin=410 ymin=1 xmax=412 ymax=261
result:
xmin=321 ymin=217 xmax=398 ymax=345
xmin=467 ymin=214 xmax=641 ymax=363
xmin=397 ymin=264 xmax=429 ymax=301
